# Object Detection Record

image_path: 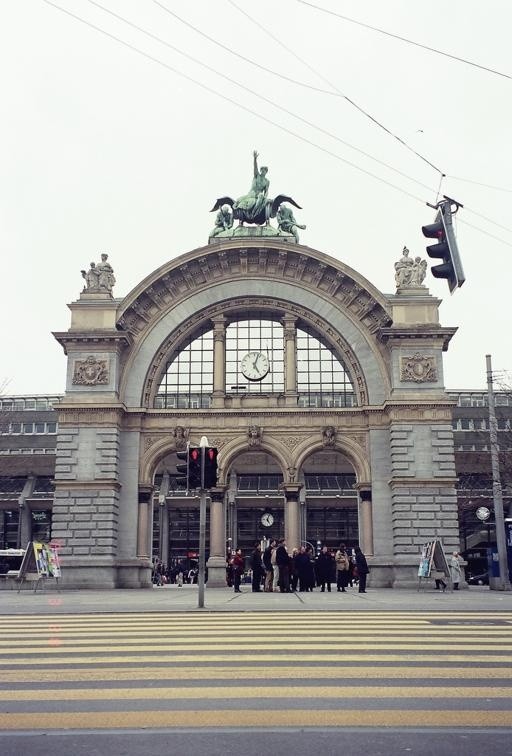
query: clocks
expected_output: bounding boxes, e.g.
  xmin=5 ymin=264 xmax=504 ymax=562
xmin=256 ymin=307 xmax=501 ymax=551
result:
xmin=241 ymin=351 xmax=270 ymax=382
xmin=261 ymin=512 xmax=274 ymax=528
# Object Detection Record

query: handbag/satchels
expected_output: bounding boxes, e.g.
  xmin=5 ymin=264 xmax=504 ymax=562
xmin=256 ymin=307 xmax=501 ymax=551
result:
xmin=232 ymin=565 xmax=240 ymax=573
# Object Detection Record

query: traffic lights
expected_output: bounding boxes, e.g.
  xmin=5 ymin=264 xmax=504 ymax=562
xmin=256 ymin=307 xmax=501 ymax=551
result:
xmin=175 ymin=445 xmax=191 ymax=493
xmin=204 ymin=446 xmax=220 ymax=489
xmin=421 ymin=201 xmax=466 ymax=297
xmin=188 ymin=446 xmax=202 ymax=489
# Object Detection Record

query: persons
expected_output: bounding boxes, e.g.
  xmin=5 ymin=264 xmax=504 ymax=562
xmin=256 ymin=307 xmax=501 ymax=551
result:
xmin=153 ymin=538 xmax=369 ymax=594
xmin=276 ymin=205 xmax=307 ymax=245
xmin=210 ymin=207 xmax=234 ymax=235
xmin=450 ymin=551 xmax=462 ymax=590
xmin=248 ymin=426 xmax=261 ymax=447
xmin=237 ymin=149 xmax=270 ymax=217
xmin=394 ymin=246 xmax=428 ymax=287
xmin=434 ymin=571 xmax=447 ymax=589
xmin=175 ymin=427 xmax=186 ymax=449
xmin=324 ymin=427 xmax=335 ymax=447
xmin=82 ymin=253 xmax=116 ymax=292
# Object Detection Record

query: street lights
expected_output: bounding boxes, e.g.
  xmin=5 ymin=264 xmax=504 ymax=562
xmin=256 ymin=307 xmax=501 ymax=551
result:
xmin=4 ymin=508 xmax=14 ymax=569
